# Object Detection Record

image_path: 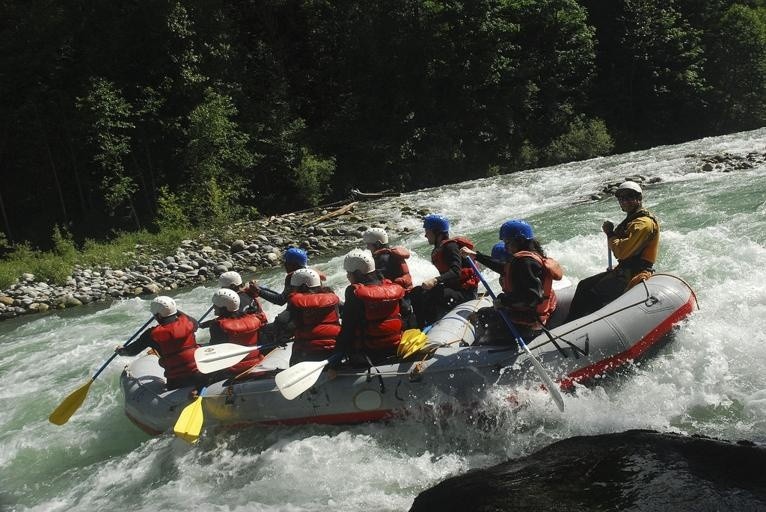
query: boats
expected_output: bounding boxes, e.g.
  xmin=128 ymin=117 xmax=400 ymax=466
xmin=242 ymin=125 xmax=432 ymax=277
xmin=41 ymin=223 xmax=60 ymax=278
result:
xmin=118 ymin=272 xmax=696 ymax=440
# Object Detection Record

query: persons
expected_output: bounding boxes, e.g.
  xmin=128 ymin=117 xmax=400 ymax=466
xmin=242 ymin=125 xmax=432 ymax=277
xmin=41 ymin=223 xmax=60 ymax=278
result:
xmin=321 ymin=247 xmax=411 ymax=380
xmin=272 ymin=267 xmax=341 ymax=379
xmin=199 ymin=270 xmax=268 ymax=336
xmin=364 ymin=226 xmax=414 ymax=295
xmin=250 ymin=246 xmax=334 ymax=335
xmin=114 ymin=296 xmax=201 ymax=390
xmin=411 ymin=214 xmax=482 ymax=324
xmin=490 ymin=242 xmax=517 ymax=292
xmin=459 ymin=220 xmax=563 ymax=345
xmin=188 ymin=289 xmax=260 ymax=383
xmin=564 ymin=180 xmax=661 ymax=327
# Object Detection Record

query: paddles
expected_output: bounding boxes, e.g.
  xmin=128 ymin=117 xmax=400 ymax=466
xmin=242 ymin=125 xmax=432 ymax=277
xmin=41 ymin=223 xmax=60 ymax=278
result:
xmin=194 ymin=340 xmax=293 ymax=376
xmin=49 ymin=313 xmax=157 ymax=425
xmin=139 ymin=301 xmax=217 ymax=356
xmin=466 ymin=254 xmax=565 ymax=414
xmin=174 ymin=374 xmax=215 ymax=445
xmin=275 ymin=354 xmax=340 ymax=402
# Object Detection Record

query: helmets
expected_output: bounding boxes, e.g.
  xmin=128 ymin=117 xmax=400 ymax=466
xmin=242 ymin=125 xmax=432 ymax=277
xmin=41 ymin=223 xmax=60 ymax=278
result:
xmin=499 ymin=220 xmax=534 ymax=240
xmin=344 ymin=249 xmax=376 ymax=274
xmin=614 ymin=181 xmax=641 ymax=194
xmin=286 ymin=249 xmax=307 ymax=266
xmin=212 ymin=289 xmax=240 ymax=310
xmin=290 ymin=269 xmax=321 ymax=287
xmin=491 ymin=243 xmax=506 ymax=260
xmin=423 ymin=215 xmax=449 ymax=232
xmin=219 ymin=272 xmax=242 ymax=287
xmin=362 ymin=228 xmax=388 ymax=244
xmin=150 ymin=296 xmax=178 ymax=316
